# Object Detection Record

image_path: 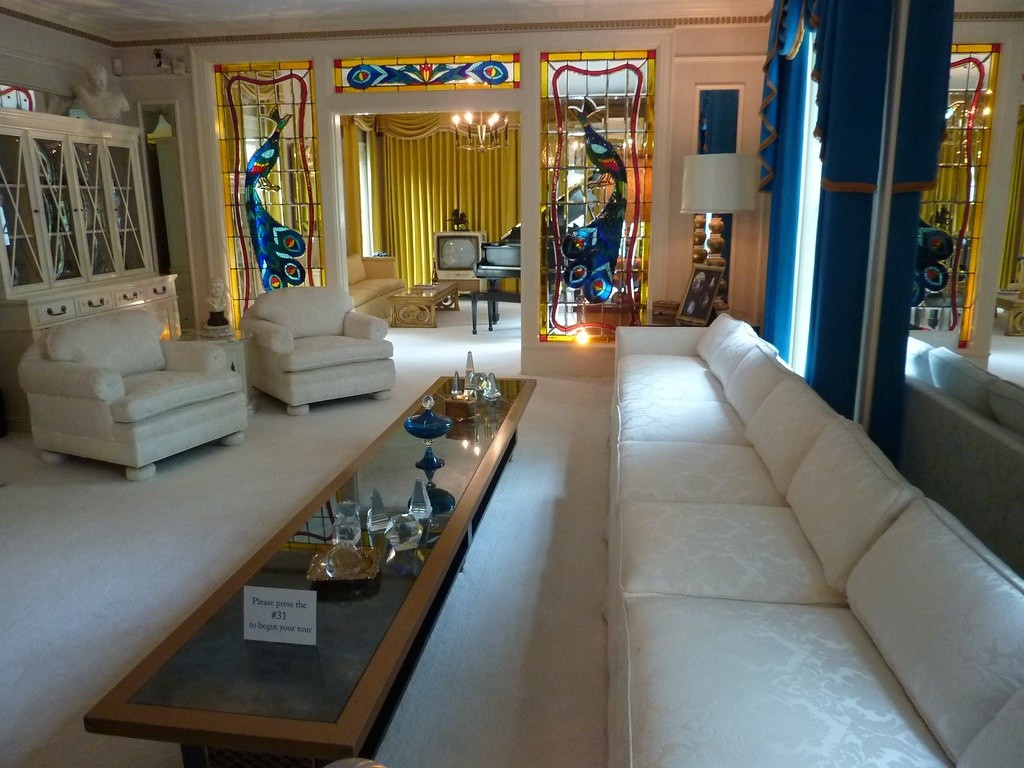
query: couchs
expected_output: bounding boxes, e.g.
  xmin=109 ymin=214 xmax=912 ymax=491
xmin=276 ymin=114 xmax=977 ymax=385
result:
xmin=611 ymin=310 xmax=1024 ymax=768
xmin=347 ymin=252 xmax=407 ymax=317
xmin=17 ymin=306 xmax=248 ymax=481
xmin=899 ymin=336 xmax=1024 ymax=581
xmin=237 ymin=285 xmax=395 ymax=416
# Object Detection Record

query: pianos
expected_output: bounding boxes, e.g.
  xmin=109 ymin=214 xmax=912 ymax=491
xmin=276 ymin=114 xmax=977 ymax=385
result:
xmin=472 ymin=166 xmax=602 ymax=334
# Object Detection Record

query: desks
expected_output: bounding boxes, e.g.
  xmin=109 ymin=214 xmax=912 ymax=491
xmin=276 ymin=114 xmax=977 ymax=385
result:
xmin=387 ymin=282 xmax=460 ymax=328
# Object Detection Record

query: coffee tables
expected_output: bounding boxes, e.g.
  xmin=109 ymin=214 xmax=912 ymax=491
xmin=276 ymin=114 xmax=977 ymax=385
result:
xmin=80 ymin=375 xmax=538 ymax=768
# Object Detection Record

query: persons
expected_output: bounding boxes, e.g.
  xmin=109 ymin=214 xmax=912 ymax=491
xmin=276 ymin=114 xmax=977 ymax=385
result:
xmin=80 ymin=63 xmax=131 ymax=118
xmin=206 ymin=277 xmax=226 ymax=312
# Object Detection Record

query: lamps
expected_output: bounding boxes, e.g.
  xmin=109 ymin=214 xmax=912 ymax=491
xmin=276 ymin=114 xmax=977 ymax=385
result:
xmin=450 ymin=110 xmax=510 ymax=154
xmin=680 ymin=153 xmax=755 ymax=309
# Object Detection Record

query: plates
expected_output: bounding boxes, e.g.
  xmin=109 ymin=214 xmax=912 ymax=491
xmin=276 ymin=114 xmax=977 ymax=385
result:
xmin=306 ymin=547 xmax=379 ymax=581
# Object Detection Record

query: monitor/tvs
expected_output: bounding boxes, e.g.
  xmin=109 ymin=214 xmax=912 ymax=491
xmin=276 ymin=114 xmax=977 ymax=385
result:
xmin=434 ymin=232 xmax=482 ymax=279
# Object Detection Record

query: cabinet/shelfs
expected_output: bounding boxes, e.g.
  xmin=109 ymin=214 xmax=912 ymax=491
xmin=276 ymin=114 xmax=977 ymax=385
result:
xmin=0 ymin=293 xmax=78 ymax=432
xmin=144 ymin=278 xmax=182 ymax=342
xmin=112 ymin=286 xmax=146 ymax=309
xmin=76 ymin=291 xmax=111 ymax=316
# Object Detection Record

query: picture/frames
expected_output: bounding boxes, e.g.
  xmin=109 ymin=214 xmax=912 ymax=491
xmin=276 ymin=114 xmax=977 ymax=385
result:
xmin=674 ymin=261 xmax=726 ymax=326
